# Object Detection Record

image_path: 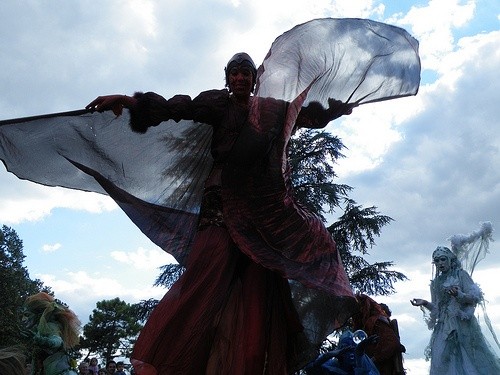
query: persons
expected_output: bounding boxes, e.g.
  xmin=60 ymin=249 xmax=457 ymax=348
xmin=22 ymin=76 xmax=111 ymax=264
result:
xmin=18 ymin=292 xmax=126 ymax=375
xmin=86 ymin=52 xmax=359 ymax=375
xmin=410 ymin=246 xmax=500 ymax=375
xmin=304 ymin=303 xmax=406 ymax=375
